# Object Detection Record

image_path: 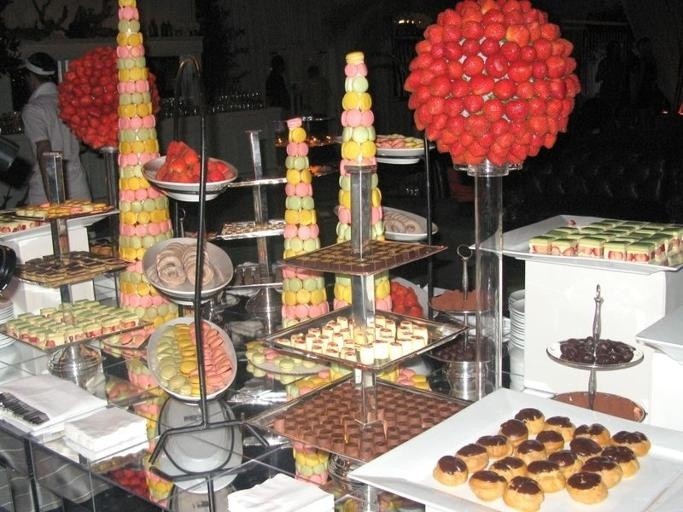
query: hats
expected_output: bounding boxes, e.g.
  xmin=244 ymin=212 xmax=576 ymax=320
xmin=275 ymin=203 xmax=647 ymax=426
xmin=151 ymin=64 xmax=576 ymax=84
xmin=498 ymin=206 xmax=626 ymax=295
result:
xmin=16 ymin=51 xmax=55 ymax=75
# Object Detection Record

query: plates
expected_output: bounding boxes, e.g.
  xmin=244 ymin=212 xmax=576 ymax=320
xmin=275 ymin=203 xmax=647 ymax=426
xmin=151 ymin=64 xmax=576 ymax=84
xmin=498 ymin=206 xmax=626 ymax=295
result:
xmin=344 ymin=386 xmax=683 ymax=510
xmin=382 ymin=203 xmax=438 ymax=243
xmin=375 ymin=147 xmax=435 ymax=165
xmin=0 ymin=207 xmax=120 ymax=244
xmin=505 ymin=289 xmax=526 ymax=355
xmin=468 ymin=211 xmax=681 ymax=274
xmin=253 ymin=303 xmax=466 ymax=370
xmin=247 ymin=370 xmax=478 ymax=464
xmin=287 ymin=234 xmax=448 ymax=282
xmin=0 ymin=300 xmax=19 ymax=349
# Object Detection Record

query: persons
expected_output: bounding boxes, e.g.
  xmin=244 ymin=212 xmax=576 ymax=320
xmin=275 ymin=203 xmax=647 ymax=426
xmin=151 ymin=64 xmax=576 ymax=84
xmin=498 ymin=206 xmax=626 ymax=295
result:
xmin=20 ymin=52 xmax=93 ymax=205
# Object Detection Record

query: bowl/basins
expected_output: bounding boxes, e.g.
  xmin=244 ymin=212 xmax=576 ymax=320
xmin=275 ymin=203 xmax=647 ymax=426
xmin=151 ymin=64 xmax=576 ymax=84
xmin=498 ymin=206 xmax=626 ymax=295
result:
xmin=137 ymin=234 xmax=235 ymax=306
xmin=146 ymin=315 xmax=236 ymax=404
xmin=140 ymin=154 xmax=238 ymax=203
xmin=151 ymin=394 xmax=243 ymax=491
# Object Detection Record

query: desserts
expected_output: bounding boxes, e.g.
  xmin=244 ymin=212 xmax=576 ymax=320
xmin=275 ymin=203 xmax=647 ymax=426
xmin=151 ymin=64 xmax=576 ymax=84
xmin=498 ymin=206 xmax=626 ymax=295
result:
xmin=1 ymin=0 xmax=683 ymax=510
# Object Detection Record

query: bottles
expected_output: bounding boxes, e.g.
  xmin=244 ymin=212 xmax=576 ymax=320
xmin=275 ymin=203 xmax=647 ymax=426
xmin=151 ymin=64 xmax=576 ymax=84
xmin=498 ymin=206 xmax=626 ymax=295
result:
xmin=149 ymin=16 xmax=197 ymax=37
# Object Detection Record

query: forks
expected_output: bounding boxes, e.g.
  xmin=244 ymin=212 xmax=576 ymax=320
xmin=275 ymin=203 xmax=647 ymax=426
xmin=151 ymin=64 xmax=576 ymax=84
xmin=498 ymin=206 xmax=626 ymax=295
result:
xmin=426 ymin=291 xmax=497 ymax=314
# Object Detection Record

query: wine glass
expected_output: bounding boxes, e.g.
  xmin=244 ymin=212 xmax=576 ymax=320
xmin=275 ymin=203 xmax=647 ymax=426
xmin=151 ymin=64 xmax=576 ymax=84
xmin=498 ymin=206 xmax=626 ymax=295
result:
xmin=157 ymin=87 xmax=265 ymax=120
